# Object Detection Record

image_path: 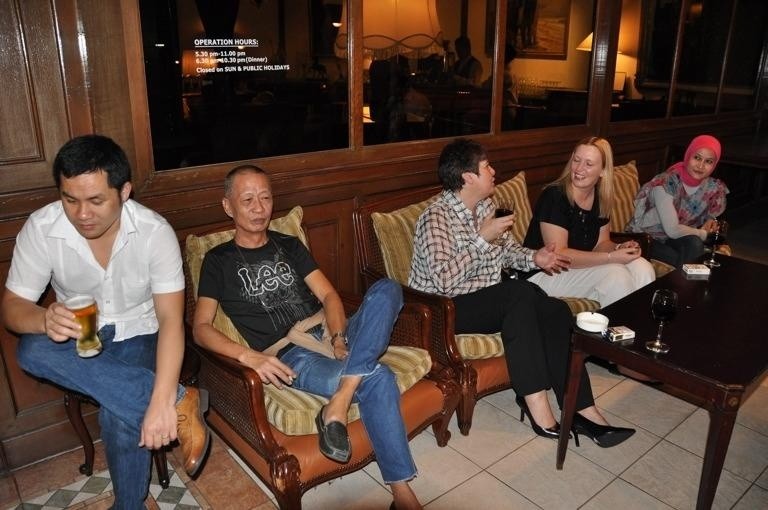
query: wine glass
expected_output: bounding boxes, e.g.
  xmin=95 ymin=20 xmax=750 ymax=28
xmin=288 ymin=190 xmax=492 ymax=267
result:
xmin=703 ymin=219 xmax=729 ymax=268
xmin=494 ymin=197 xmax=515 ymax=245
xmin=646 ymin=289 xmax=680 ymax=352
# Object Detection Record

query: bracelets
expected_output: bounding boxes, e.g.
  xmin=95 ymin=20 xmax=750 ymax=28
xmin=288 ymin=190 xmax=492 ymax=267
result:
xmin=614 ymin=243 xmax=623 ymax=250
xmin=331 ymin=331 xmax=346 ymax=345
xmin=607 ymin=251 xmax=612 ymax=264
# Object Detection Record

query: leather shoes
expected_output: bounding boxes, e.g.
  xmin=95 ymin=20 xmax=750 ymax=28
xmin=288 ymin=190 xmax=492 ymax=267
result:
xmin=174 ymin=385 xmax=212 ymax=479
xmin=314 ymin=406 xmax=351 ymax=464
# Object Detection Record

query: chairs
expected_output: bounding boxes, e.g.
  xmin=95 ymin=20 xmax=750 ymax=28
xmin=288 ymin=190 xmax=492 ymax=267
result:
xmin=608 ymin=214 xmax=730 ymax=263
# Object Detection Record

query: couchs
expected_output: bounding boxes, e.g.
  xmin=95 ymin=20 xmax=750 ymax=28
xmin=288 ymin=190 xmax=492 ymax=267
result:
xmin=179 ymin=218 xmax=461 ymax=509
xmin=350 ymin=185 xmax=594 ymax=434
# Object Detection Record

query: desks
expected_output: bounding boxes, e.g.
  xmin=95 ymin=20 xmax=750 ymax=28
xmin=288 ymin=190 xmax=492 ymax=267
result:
xmin=661 ymin=138 xmax=768 ymax=201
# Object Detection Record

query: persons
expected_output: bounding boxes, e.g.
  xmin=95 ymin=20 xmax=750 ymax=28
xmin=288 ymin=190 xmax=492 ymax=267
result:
xmin=191 ymin=165 xmax=424 ymax=510
xmin=386 ymin=76 xmax=433 ymax=141
xmin=517 ymin=135 xmax=657 ymax=309
xmin=470 ymin=41 xmax=520 ymax=131
xmin=1 ymin=133 xmax=212 ymax=510
xmin=623 ymin=135 xmax=733 ymax=271
xmin=441 ymin=35 xmax=483 ymax=89
xmin=406 ymin=137 xmax=636 ymax=448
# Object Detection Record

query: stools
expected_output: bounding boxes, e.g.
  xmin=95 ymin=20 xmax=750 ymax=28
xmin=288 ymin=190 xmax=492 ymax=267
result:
xmin=64 ymin=320 xmax=199 ymax=489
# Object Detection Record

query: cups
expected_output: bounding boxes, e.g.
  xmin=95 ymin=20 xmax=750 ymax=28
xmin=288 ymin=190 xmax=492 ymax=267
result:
xmin=65 ymin=294 xmax=103 ymax=358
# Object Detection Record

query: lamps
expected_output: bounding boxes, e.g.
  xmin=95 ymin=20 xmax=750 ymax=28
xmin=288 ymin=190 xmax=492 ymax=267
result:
xmin=575 ymin=30 xmax=594 ymax=52
xmin=333 ymin=1 xmax=446 ymax=61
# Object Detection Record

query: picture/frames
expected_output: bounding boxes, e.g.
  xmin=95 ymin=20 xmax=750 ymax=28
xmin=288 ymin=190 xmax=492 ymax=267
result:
xmin=484 ymin=0 xmax=571 ymax=60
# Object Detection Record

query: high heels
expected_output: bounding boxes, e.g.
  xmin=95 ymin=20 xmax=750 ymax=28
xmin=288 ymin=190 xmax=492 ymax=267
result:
xmin=514 ymin=393 xmax=574 ymax=439
xmin=572 ymin=411 xmax=637 ymax=450
